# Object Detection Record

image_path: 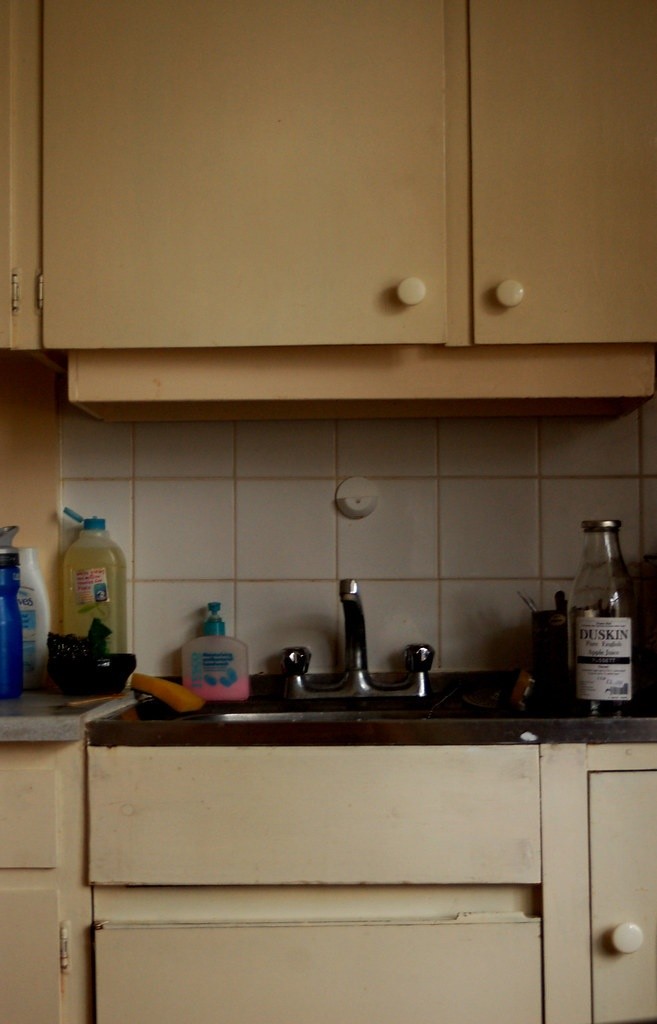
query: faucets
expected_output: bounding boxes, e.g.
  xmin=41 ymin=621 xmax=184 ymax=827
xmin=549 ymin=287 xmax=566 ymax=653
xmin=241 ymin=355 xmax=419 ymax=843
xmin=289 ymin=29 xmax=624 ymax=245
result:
xmin=335 ymin=576 xmax=370 ymax=698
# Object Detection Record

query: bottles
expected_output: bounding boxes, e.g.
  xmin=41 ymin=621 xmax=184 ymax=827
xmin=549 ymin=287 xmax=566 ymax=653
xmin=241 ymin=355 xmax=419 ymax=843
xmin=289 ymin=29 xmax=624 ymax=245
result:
xmin=62 ymin=507 xmax=126 ymax=652
xmin=0 ymin=524 xmax=24 ymax=701
xmin=567 ymin=521 xmax=633 ymax=716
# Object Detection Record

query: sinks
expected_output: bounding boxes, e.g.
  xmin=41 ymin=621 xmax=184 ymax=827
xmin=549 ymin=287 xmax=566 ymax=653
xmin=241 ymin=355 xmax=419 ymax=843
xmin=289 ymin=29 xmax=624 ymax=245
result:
xmin=188 ymin=709 xmax=460 ymax=726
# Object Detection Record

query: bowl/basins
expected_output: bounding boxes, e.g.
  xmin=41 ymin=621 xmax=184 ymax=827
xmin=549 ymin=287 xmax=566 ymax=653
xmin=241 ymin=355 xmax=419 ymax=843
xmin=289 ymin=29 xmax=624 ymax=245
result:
xmin=47 ymin=654 xmax=136 ymax=697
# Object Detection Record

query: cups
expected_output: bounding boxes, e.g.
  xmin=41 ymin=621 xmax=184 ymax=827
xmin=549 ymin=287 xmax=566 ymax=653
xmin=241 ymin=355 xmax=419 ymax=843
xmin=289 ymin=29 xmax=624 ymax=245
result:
xmin=531 ymin=609 xmax=568 ymax=667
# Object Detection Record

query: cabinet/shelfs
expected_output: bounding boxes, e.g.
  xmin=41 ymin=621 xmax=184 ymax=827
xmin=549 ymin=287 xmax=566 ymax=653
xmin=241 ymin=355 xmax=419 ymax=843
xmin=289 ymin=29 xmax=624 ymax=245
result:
xmin=0 ymin=1 xmax=657 ymax=420
xmin=0 ymin=745 xmax=657 ymax=1024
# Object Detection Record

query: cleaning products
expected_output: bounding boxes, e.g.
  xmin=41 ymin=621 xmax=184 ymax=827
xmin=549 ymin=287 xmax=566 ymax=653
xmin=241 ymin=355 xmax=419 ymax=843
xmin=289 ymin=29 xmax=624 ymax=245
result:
xmin=179 ymin=599 xmax=252 ymax=703
xmin=2 ymin=507 xmax=129 ymax=701
